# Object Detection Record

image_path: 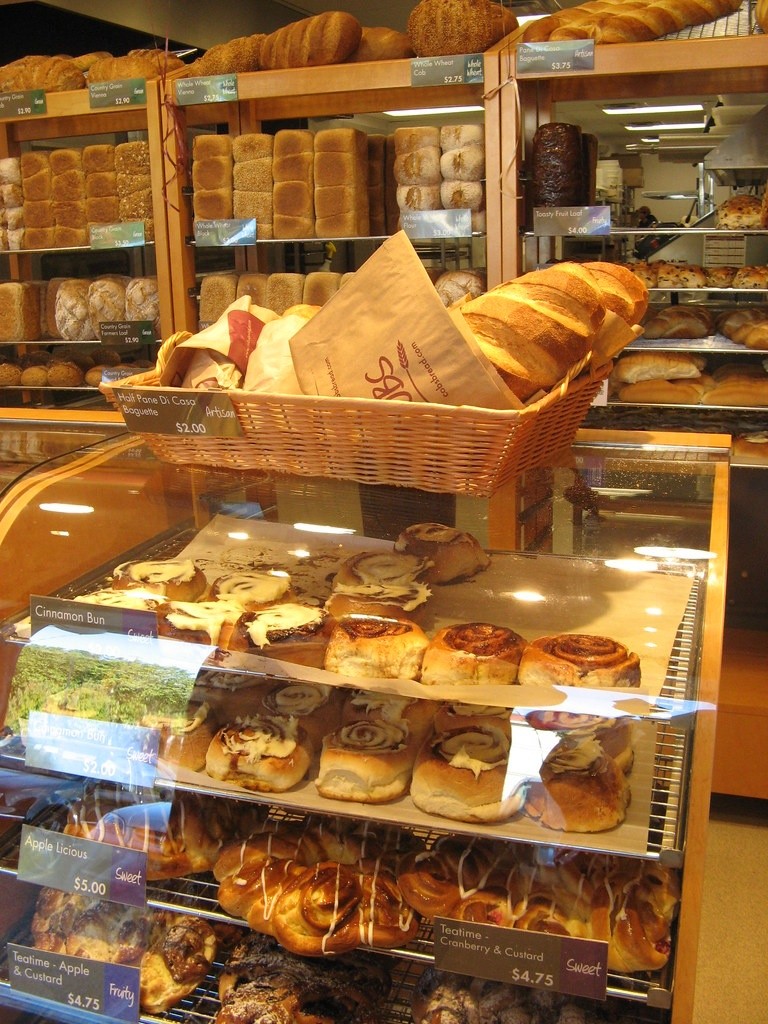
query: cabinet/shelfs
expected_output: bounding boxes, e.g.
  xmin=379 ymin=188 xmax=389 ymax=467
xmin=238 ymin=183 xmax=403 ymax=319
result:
xmin=0 ymin=28 xmax=768 ymax=487
xmin=2 ymin=429 xmax=729 ymax=1024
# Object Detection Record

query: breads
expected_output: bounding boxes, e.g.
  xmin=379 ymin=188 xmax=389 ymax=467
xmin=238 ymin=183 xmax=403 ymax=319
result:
xmin=1 ymin=0 xmax=519 ymax=340
xmin=523 ymin=0 xmax=768 ymax=463
xmin=0 ymin=346 xmax=163 ymax=386
xmin=450 ymin=260 xmax=651 ymax=403
xmin=29 ymin=519 xmax=680 ymax=1024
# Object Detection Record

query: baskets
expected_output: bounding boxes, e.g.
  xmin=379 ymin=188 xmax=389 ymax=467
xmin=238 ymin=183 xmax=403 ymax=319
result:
xmin=99 ymin=331 xmax=612 ymax=499
